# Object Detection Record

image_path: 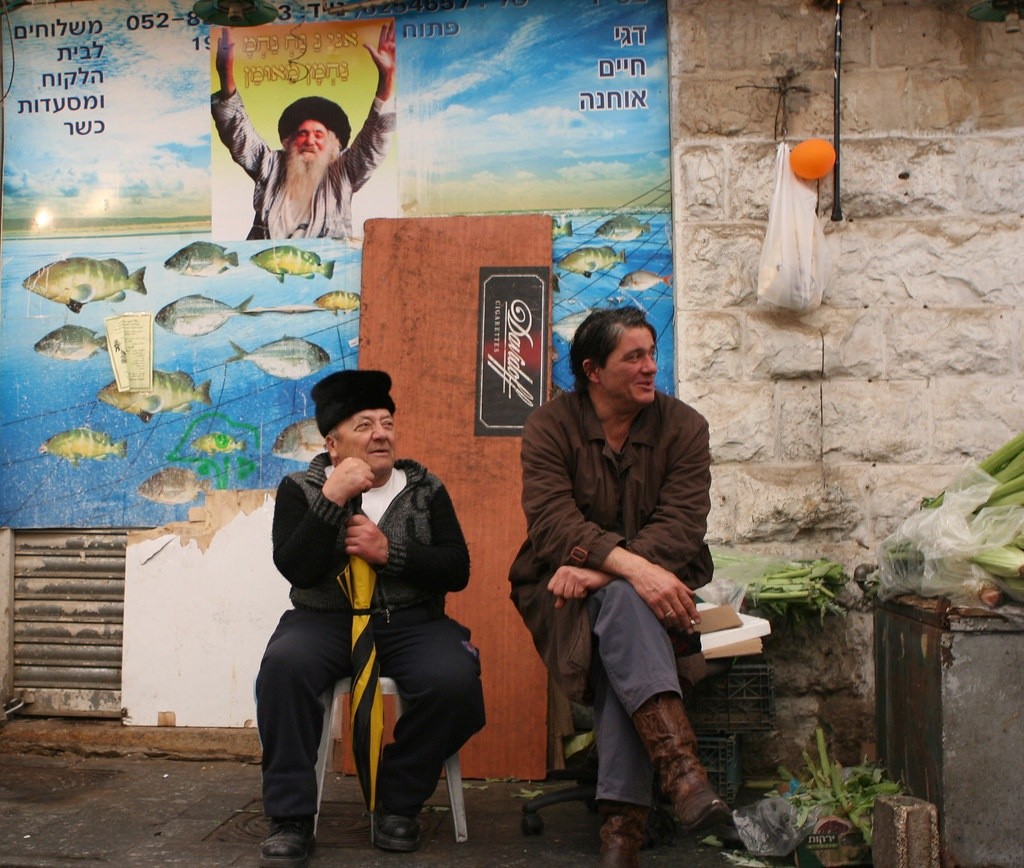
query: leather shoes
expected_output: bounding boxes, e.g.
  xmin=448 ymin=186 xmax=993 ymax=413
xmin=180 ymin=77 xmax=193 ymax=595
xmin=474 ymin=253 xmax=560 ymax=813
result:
xmin=373 ymin=811 xmax=420 ymax=852
xmin=260 ymin=817 xmax=316 ymax=868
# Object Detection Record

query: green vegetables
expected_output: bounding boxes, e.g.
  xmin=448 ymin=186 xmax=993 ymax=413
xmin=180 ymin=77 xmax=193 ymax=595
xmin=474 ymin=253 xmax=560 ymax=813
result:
xmin=865 ymin=428 xmax=1024 ymax=608
xmin=763 ymin=725 xmax=911 ymax=845
xmin=712 ymin=552 xmax=847 ymax=627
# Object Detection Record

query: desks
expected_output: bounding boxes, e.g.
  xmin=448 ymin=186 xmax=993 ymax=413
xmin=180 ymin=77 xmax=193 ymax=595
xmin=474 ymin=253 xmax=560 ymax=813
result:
xmin=870 ymin=583 xmax=1024 ymax=868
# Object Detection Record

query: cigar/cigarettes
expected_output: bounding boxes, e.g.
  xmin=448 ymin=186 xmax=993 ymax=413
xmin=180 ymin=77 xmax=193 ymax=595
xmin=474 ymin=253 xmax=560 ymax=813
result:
xmin=689 ymin=617 xmax=696 ymax=625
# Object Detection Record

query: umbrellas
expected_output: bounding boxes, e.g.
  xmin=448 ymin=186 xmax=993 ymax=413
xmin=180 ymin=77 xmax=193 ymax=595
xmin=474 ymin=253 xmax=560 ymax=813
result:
xmin=336 ymin=488 xmax=386 ymax=849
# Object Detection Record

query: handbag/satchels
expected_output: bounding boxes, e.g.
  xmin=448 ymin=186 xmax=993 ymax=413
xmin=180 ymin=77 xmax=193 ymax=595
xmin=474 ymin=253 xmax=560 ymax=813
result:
xmin=757 ymin=144 xmax=826 ymax=314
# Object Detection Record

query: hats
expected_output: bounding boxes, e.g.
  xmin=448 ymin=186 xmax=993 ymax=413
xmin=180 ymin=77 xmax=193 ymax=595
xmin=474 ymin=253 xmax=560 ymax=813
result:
xmin=311 ymin=369 xmax=395 ymax=438
xmin=278 ymin=95 xmax=350 ymax=152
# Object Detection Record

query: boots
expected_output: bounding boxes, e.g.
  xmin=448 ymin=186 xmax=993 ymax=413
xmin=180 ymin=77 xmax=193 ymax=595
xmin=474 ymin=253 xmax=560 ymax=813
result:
xmin=599 ymin=803 xmax=644 ymax=868
xmin=632 ymin=695 xmax=729 ymax=837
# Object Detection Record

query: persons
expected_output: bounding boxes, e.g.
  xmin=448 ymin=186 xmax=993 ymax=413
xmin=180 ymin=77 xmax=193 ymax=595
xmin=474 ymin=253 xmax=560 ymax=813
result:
xmin=256 ymin=370 xmax=487 ymax=867
xmin=211 ymin=21 xmax=395 ymax=240
xmin=508 ymin=306 xmax=735 ymax=868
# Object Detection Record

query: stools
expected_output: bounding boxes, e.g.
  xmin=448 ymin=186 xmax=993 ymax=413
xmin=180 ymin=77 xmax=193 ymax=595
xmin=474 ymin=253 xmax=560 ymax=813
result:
xmin=313 ymin=673 xmax=468 ymax=843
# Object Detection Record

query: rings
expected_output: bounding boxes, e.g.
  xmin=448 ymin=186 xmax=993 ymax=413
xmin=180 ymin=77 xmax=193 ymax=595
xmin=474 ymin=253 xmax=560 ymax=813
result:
xmin=665 ymin=609 xmax=673 ymax=617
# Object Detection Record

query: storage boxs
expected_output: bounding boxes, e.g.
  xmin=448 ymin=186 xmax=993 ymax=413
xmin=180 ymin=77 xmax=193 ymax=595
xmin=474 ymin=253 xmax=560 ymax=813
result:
xmin=773 ymin=783 xmax=872 ymax=868
xmin=683 ymin=658 xmax=776 ymax=734
xmin=652 ymin=734 xmax=744 ymax=805
